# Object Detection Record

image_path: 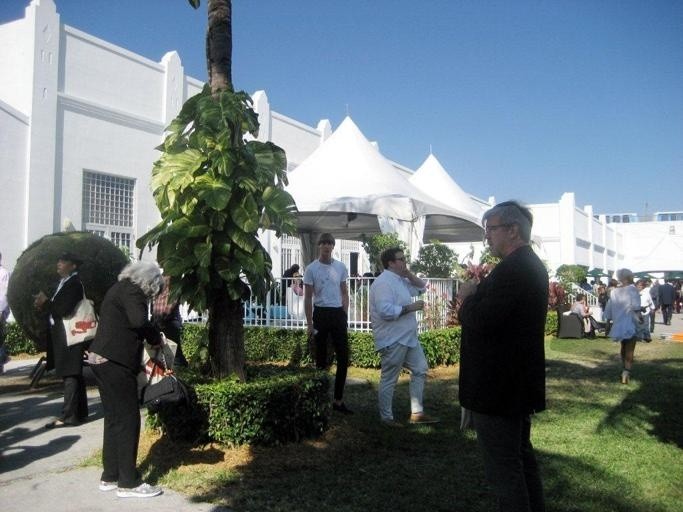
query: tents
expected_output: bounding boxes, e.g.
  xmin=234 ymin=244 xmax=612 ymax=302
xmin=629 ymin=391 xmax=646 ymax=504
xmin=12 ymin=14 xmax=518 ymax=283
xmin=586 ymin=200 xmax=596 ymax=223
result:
xmin=259 ymin=117 xmax=486 ymax=268
xmin=408 ymin=143 xmax=489 ymax=225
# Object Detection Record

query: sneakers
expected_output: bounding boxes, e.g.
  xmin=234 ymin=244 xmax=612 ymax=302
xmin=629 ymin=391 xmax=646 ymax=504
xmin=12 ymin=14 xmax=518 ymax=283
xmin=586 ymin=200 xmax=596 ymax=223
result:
xmin=100 ymin=476 xmax=118 ymax=491
xmin=117 ymin=479 xmax=164 ymax=497
xmin=410 ymin=413 xmax=441 ymax=424
xmin=380 ymin=417 xmax=403 ymax=431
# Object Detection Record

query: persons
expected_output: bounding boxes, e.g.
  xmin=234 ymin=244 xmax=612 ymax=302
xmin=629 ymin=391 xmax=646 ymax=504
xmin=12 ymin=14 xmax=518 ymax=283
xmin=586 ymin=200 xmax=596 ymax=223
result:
xmin=636 ymin=280 xmax=655 ymax=343
xmin=282 ymin=264 xmax=302 ymax=304
xmin=151 ymin=270 xmax=188 ymax=369
xmin=643 ymin=278 xmax=658 ymax=333
xmin=604 ymin=279 xmax=618 ymax=337
xmin=88 ymin=258 xmax=167 ymax=498
xmin=593 ymin=281 xmax=606 ymax=296
xmin=359 ymin=272 xmax=373 ymax=290
xmin=453 ymin=200 xmax=548 ymax=511
xmin=602 ymin=269 xmax=644 ymax=384
xmin=666 ymin=279 xmax=682 ymax=313
xmin=649 ymin=282 xmax=659 ymax=311
xmin=32 ymin=250 xmax=94 ymax=429
xmin=571 ymin=294 xmax=606 ymax=339
xmin=1 ymin=252 xmax=12 ymax=376
xmin=599 ymin=287 xmax=610 ymax=322
xmin=302 ymin=233 xmax=350 ymax=414
xmin=659 ymin=279 xmax=676 ymax=325
xmin=369 ymin=248 xmax=442 ymax=428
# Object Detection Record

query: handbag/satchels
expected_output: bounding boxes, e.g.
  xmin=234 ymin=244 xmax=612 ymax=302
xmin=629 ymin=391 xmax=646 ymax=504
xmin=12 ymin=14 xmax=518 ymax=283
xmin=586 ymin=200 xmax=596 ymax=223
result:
xmin=142 ymin=344 xmax=186 ymax=415
xmin=60 ymin=280 xmax=98 ymax=347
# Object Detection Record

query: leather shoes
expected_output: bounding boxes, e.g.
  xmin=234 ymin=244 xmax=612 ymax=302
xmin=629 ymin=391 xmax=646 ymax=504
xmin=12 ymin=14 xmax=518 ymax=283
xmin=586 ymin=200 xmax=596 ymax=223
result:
xmin=44 ymin=416 xmax=80 ymax=429
xmin=333 ymin=401 xmax=353 ymax=414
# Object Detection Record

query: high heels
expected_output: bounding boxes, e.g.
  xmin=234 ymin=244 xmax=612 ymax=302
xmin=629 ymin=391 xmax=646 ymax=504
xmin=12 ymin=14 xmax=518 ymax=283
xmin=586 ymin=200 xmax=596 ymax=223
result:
xmin=621 ymin=370 xmax=631 ymax=384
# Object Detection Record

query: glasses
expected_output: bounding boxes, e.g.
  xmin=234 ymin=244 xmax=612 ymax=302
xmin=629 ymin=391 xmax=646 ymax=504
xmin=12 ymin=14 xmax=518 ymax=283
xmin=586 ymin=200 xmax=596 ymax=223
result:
xmin=486 ymin=223 xmax=508 ymax=231
xmin=390 ymin=255 xmax=406 ymax=263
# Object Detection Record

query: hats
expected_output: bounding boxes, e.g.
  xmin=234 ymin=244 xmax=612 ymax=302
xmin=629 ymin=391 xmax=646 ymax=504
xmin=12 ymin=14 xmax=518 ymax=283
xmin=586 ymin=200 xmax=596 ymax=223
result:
xmin=56 ymin=249 xmax=82 ymax=263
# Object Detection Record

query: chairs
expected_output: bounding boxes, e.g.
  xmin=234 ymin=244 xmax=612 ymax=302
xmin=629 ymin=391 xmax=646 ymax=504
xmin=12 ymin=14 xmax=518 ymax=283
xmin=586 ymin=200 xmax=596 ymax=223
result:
xmin=557 ymin=305 xmax=583 ymax=339
xmin=30 ymin=350 xmax=90 ymax=388
